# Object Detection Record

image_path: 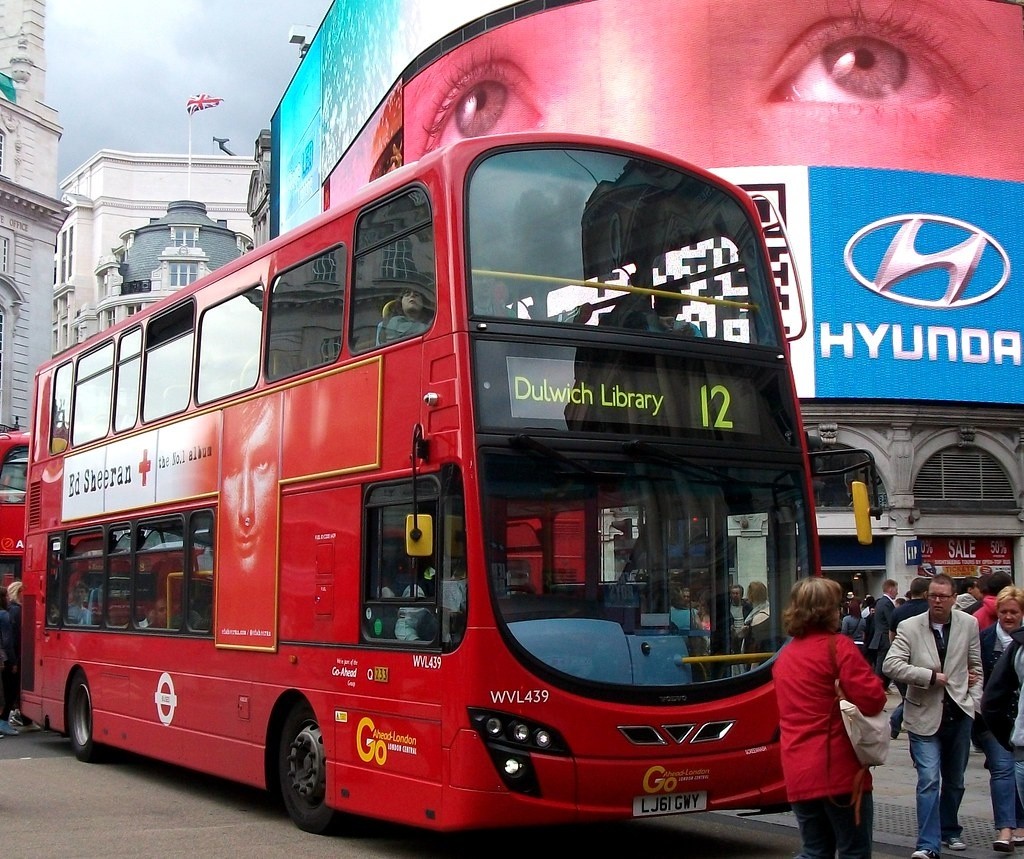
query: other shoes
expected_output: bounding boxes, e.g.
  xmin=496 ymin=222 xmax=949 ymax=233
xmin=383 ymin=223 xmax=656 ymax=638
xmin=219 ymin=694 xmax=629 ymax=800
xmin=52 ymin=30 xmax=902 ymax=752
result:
xmin=0 ymin=720 xmax=18 ymax=735
xmin=993 ymin=840 xmax=1014 ymax=852
xmin=1010 ymin=836 xmax=1024 ymax=846
xmin=24 ymin=725 xmax=40 ymax=731
xmin=972 ymin=742 xmax=982 ymax=752
xmin=889 ymin=721 xmax=899 ymax=740
xmin=12 ymin=716 xmax=23 ymax=725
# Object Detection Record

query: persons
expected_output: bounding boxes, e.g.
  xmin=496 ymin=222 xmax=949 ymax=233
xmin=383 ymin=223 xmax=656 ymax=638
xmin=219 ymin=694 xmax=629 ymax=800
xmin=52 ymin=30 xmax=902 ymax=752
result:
xmin=747 ymin=581 xmax=770 ymax=672
xmin=773 ymin=578 xmax=888 ymax=859
xmin=883 ymin=575 xmax=983 ymax=859
xmin=0 ymin=580 xmax=42 ymax=738
xmin=50 ymin=277 xmax=730 ymax=697
xmin=973 ymin=571 xmax=1013 ymax=632
xmin=868 ymin=579 xmax=899 ymax=695
xmin=888 ymin=577 xmax=930 ymax=739
xmin=403 ymin=0 xmax=1024 ymax=181
xmin=221 ymin=394 xmax=281 ymax=578
xmin=864 ymin=603 xmax=877 ymax=669
xmin=841 ymin=601 xmax=866 ymax=660
xmin=841 ymin=577 xmax=986 ymax=625
xmin=980 ymin=625 xmax=1024 ymax=807
xmin=728 ymin=585 xmax=753 ymax=676
xmin=968 ymin=586 xmax=1024 ymax=853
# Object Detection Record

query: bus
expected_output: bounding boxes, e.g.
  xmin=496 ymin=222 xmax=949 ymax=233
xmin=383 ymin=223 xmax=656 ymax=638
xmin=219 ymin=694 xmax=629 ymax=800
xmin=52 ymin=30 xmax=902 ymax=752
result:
xmin=1 ymin=429 xmax=30 ymax=595
xmin=16 ymin=140 xmax=884 ymax=832
xmin=72 ymin=505 xmax=586 ymax=630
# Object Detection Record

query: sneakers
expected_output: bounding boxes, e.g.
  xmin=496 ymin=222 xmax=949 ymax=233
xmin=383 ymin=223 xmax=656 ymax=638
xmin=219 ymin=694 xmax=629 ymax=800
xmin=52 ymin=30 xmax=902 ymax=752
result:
xmin=911 ymin=849 xmax=940 ymax=859
xmin=948 ymin=837 xmax=966 ymax=849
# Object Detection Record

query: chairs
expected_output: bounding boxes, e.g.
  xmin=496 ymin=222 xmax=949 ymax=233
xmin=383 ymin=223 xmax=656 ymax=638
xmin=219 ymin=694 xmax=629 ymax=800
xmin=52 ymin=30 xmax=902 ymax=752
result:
xmin=377 ymin=300 xmax=399 ymax=347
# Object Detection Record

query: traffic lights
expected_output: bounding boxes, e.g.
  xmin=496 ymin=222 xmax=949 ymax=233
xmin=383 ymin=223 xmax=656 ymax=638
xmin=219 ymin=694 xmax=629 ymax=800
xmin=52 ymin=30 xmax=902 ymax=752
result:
xmin=612 ymin=517 xmax=632 ymax=541
xmin=688 ymin=514 xmax=706 ymax=545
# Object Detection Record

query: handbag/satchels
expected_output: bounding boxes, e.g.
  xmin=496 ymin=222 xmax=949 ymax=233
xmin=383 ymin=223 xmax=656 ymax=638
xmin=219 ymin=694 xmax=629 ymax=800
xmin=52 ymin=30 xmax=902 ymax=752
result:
xmin=830 ymin=635 xmax=891 ymax=766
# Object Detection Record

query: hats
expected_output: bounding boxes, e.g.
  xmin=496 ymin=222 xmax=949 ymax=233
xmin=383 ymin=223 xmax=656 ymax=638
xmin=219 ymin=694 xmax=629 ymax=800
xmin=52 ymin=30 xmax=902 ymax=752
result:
xmin=847 ymin=592 xmax=854 ymax=598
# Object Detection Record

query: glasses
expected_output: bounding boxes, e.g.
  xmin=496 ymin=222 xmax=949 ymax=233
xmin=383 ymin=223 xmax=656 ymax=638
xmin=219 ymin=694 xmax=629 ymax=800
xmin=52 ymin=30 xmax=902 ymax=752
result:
xmin=927 ymin=592 xmax=956 ymax=602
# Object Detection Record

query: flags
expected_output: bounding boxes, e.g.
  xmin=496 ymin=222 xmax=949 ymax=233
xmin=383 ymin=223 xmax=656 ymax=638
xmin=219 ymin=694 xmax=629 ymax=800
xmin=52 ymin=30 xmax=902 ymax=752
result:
xmin=187 ymin=93 xmax=224 ymax=115
